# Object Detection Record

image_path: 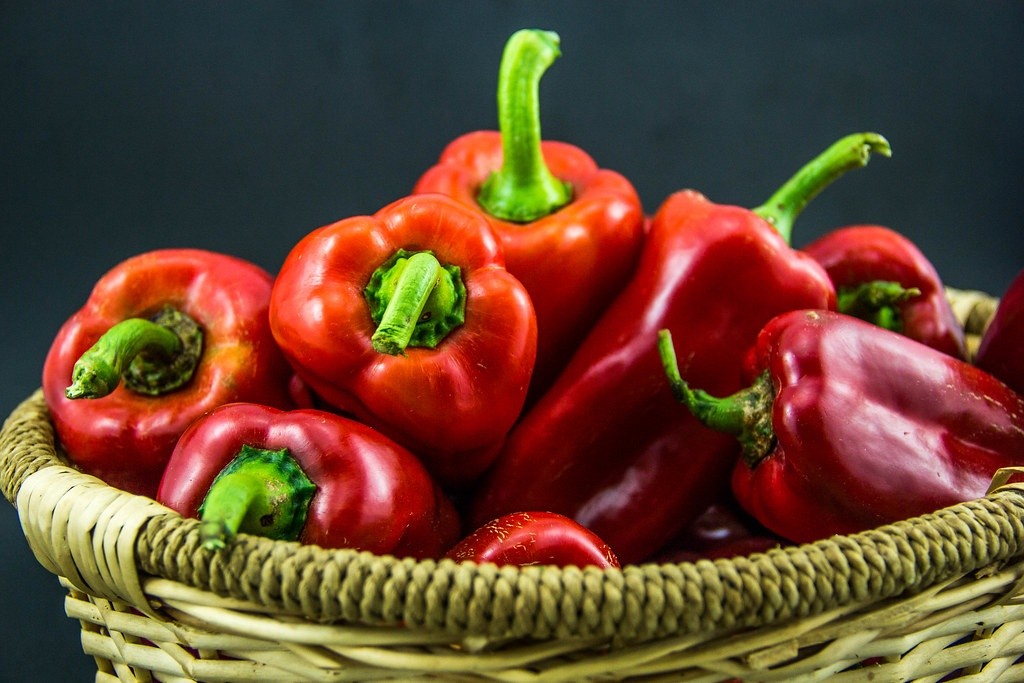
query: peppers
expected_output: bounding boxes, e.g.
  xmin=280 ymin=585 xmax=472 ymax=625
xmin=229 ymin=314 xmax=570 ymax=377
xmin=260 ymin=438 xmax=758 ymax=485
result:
xmin=41 ymin=27 xmax=1024 ymax=563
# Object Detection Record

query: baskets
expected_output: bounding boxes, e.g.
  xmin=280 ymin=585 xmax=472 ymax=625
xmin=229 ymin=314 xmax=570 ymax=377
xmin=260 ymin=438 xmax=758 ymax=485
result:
xmin=0 ymin=288 xmax=1024 ymax=683
